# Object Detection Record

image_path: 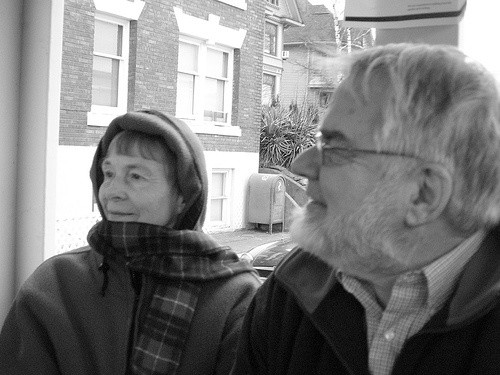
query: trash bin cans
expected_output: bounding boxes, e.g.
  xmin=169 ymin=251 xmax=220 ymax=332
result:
xmin=247 ymin=173 xmax=286 ymax=234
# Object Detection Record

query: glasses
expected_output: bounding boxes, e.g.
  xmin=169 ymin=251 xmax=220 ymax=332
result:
xmin=313 ymin=131 xmax=424 ymax=165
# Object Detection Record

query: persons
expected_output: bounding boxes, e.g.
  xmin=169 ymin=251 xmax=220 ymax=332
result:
xmin=232 ymin=42 xmax=500 ymax=375
xmin=0 ymin=107 xmax=264 ymax=375
